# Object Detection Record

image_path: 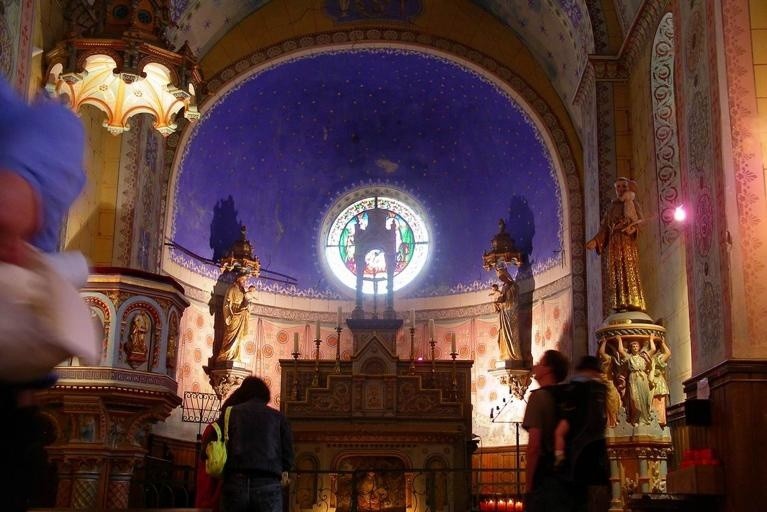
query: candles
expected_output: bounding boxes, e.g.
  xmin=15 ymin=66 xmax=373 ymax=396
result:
xmin=316 ymin=319 xmax=320 ymax=339
xmin=410 ymin=308 xmax=415 ymax=328
xmin=337 ymin=305 xmax=342 ymax=326
xmin=452 ymin=332 xmax=456 ymax=353
xmin=294 ymin=332 xmax=299 ymax=352
xmin=429 ymin=318 xmax=434 ymax=337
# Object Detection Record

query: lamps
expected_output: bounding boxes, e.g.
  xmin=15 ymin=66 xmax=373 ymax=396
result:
xmin=40 ymin=0 xmax=206 ymax=138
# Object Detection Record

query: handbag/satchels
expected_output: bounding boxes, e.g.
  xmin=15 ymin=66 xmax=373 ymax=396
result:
xmin=205 ymin=405 xmax=233 ymax=477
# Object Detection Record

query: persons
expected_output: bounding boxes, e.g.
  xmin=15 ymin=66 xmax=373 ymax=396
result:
xmin=549 ymin=355 xmax=613 ymax=512
xmin=584 ymin=174 xmax=648 ymax=311
xmin=122 ymin=307 xmax=150 ymax=355
xmin=486 ymin=263 xmax=525 ymax=362
xmin=214 ymin=272 xmax=252 ymax=362
xmin=217 ymin=374 xmax=296 ymax=512
xmin=520 ymin=350 xmax=570 ymax=510
xmin=0 ymin=71 xmax=90 ymax=512
xmin=244 ymin=285 xmax=259 ymax=305
xmin=596 ymin=330 xmax=672 ymax=431
xmin=192 ymin=415 xmax=228 ymax=511
xmin=615 ymin=181 xmax=637 ymax=224
